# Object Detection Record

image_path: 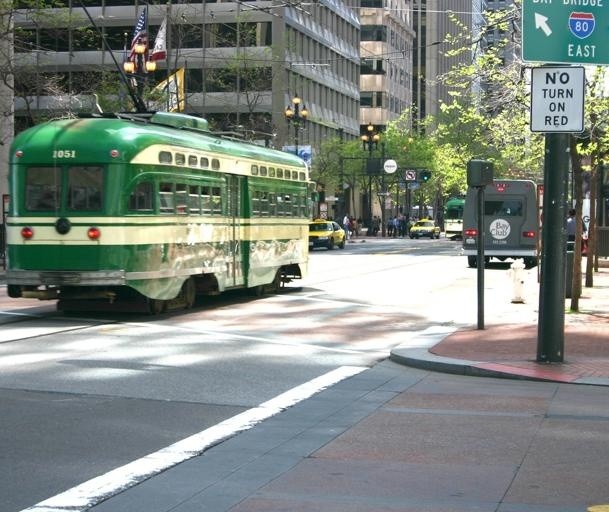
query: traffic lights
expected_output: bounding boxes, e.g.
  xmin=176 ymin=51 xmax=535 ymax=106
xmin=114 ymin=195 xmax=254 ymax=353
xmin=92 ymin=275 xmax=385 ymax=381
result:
xmin=466 ymin=157 xmax=494 ymax=188
xmin=423 ymin=170 xmax=428 ymax=181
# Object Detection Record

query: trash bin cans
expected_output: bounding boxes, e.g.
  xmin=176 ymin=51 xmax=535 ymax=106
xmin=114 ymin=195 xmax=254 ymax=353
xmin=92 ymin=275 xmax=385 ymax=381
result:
xmin=566 ymin=241 xmax=582 ymax=298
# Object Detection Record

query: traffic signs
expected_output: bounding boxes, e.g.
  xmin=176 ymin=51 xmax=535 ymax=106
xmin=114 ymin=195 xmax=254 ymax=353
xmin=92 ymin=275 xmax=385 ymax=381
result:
xmin=530 ymin=65 xmax=584 ymax=134
xmin=521 ymin=0 xmax=609 ymax=64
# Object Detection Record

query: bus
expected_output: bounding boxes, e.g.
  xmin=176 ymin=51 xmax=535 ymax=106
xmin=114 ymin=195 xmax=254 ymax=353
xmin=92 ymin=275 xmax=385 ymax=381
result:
xmin=0 ymin=109 xmax=316 ymax=319
xmin=443 ymin=194 xmax=465 ymax=240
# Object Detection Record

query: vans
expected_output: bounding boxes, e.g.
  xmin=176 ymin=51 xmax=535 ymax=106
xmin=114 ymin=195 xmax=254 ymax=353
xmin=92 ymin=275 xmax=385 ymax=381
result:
xmin=463 ymin=177 xmax=539 ymax=268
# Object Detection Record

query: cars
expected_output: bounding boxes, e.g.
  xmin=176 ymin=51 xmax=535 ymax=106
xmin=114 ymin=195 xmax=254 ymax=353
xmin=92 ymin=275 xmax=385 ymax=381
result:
xmin=309 ymin=218 xmax=346 ymax=253
xmin=409 ymin=219 xmax=440 ymax=240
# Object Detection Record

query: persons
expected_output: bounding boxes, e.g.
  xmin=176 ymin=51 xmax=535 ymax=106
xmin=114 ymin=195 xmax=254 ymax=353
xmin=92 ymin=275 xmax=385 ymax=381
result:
xmin=566 ymin=209 xmax=577 ymax=253
xmin=327 ymin=213 xmax=432 ymax=240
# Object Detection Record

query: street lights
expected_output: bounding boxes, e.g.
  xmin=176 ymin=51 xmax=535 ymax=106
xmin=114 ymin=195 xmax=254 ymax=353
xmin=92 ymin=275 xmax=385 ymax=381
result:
xmin=285 ymin=91 xmax=308 ymax=154
xmin=360 ymin=122 xmax=379 ymax=235
xmin=122 ymin=38 xmax=156 ymax=112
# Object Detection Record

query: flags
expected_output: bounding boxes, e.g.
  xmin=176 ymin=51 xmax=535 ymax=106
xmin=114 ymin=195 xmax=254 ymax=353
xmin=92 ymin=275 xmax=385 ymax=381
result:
xmin=129 ymin=8 xmax=148 ymax=88
xmin=129 ymin=67 xmax=184 ymax=113
xmin=116 ymin=43 xmax=127 ymax=113
xmin=149 ymin=15 xmax=167 ymax=62
